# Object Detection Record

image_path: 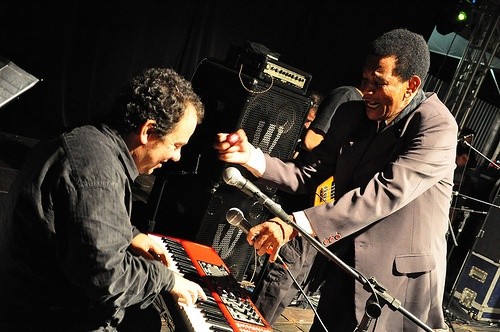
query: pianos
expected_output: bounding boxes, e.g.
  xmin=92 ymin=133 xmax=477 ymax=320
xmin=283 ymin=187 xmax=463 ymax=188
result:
xmin=146 ymin=232 xmax=274 ymax=332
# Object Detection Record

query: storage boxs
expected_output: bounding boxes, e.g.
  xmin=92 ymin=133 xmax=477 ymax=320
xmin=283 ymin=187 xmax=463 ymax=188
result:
xmin=451 ymin=251 xmax=500 ymax=322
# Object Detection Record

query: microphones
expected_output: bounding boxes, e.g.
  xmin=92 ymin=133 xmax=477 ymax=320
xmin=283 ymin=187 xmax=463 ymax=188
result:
xmin=225 ymin=208 xmax=273 ymax=255
xmin=221 ymin=167 xmax=293 ymax=225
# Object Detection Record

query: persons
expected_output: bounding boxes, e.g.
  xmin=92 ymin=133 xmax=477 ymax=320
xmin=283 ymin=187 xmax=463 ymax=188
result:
xmin=0 ymin=64 xmax=208 ymax=332
xmin=214 ymin=29 xmax=477 ymax=332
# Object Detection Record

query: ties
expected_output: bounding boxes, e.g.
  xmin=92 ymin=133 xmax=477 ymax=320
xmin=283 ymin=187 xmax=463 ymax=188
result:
xmin=378 ymin=120 xmax=386 ymax=133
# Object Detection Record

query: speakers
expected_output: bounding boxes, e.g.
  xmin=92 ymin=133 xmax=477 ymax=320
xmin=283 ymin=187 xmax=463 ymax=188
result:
xmin=137 ymin=166 xmax=277 ymax=284
xmin=181 ymin=55 xmax=315 ymax=193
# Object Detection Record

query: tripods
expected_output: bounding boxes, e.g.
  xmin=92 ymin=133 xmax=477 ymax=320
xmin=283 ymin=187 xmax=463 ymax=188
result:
xmin=443 ymin=186 xmax=500 ymax=332
